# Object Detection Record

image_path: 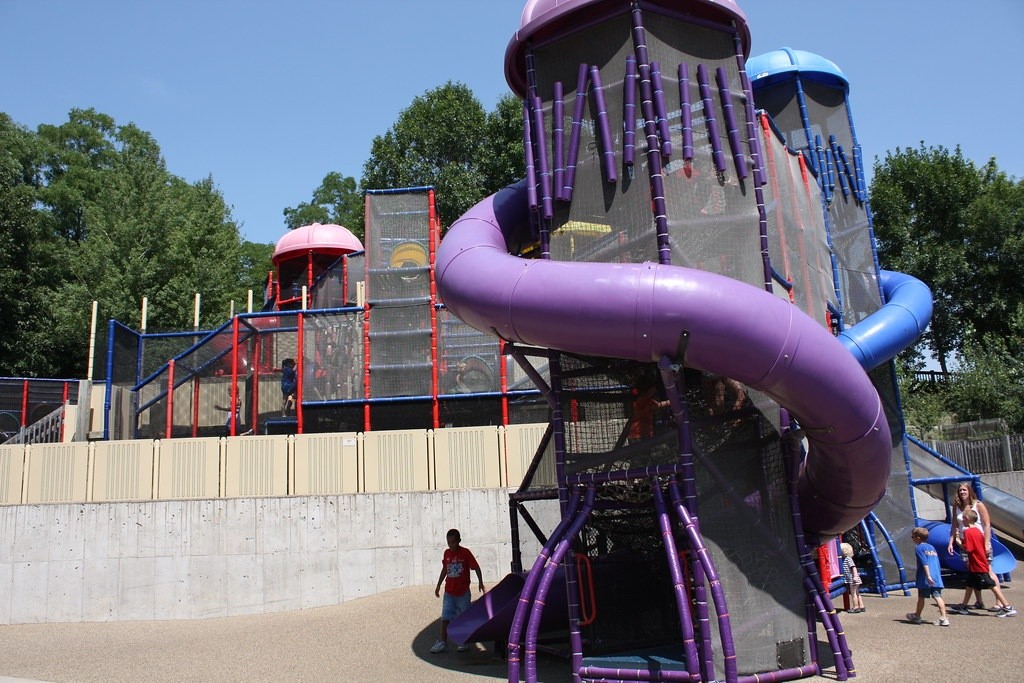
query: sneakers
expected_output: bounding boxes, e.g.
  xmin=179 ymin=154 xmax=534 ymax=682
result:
xmin=933 ymin=617 xmax=949 ymax=626
xmin=906 ymin=613 xmax=921 ymax=623
xmin=995 ymin=605 xmax=1016 ymax=617
xmin=430 ymin=640 xmax=449 ymax=654
xmin=457 ymin=642 xmax=471 ymax=652
xmin=951 ymin=603 xmax=969 ymax=614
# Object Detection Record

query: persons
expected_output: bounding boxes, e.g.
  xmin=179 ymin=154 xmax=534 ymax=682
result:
xmin=213 ymin=385 xmax=242 ymax=436
xmin=446 ymin=361 xmax=467 ymax=394
xmin=906 ymin=527 xmax=951 ymax=627
xmin=700 ymin=369 xmax=748 ymax=429
xmin=280 ymin=358 xmax=298 ymax=418
xmin=948 ymin=482 xmax=1003 ymax=612
xmin=293 ymin=355 xmax=323 ymax=401
xmin=839 ymin=543 xmax=865 ymax=613
xmin=430 ymin=529 xmax=486 ymax=651
xmin=949 ymin=510 xmax=1017 ymax=617
xmin=694 ymin=163 xmax=735 ymax=277
xmin=323 ymin=342 xmax=347 ymax=400
xmin=623 ymin=376 xmax=671 ymax=489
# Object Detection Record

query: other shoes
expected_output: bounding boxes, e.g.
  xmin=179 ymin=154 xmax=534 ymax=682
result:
xmin=969 ymin=602 xmax=985 ymax=610
xmin=988 ymin=604 xmax=1004 ymax=612
xmin=859 ymin=607 xmax=866 ymax=612
xmin=847 ymin=608 xmax=860 ymax=613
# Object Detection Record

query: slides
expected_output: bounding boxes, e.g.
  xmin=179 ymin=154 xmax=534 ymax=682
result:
xmin=433 ymin=171 xmax=1024 ymax=646
xmin=214 ymin=297 xmax=282 ymax=373
